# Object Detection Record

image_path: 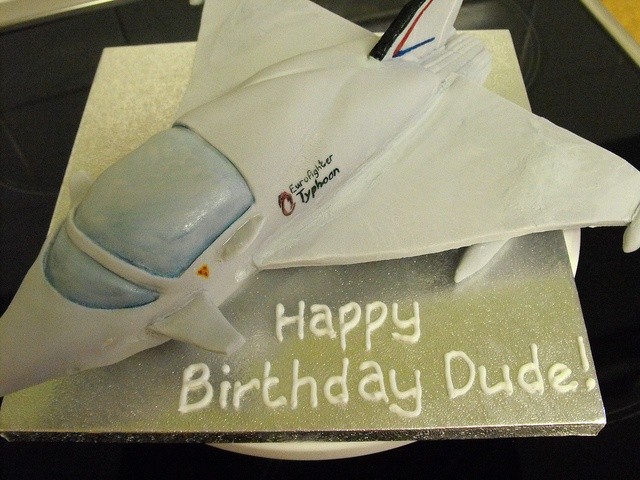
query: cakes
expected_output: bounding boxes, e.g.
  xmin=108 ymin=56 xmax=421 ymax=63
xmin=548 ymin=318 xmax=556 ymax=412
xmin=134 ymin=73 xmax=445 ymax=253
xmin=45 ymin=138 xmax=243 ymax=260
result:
xmin=1 ymin=2 xmax=640 ymax=396
